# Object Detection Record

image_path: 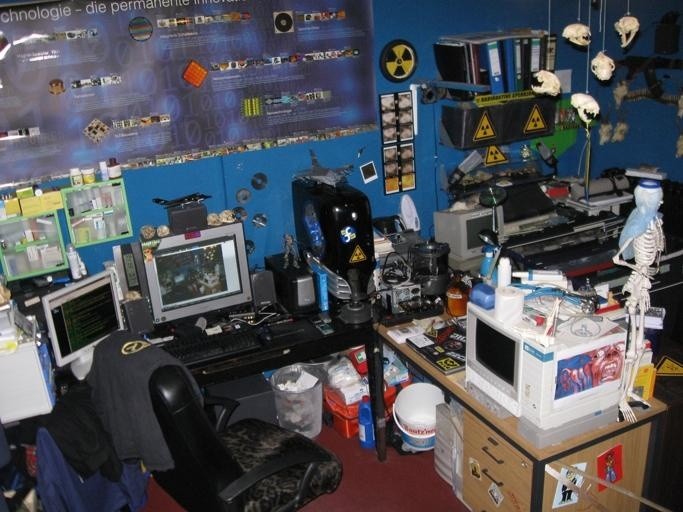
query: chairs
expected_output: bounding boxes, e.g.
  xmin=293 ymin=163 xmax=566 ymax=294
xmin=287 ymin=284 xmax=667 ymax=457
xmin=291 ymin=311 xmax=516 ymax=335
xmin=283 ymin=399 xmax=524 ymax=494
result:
xmin=87 ymin=331 xmax=343 ymax=511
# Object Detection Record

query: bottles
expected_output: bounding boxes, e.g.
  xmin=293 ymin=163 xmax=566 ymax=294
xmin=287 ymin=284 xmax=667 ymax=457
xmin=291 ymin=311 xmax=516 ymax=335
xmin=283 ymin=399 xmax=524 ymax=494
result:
xmin=356 ymin=396 xmax=373 ymax=451
xmin=98 ymin=161 xmax=108 ymax=183
xmin=479 ymin=245 xmax=496 ymax=289
xmin=496 ymin=257 xmax=511 ymax=289
xmin=63 ymin=243 xmax=82 ymax=282
xmin=80 ymin=166 xmax=97 ymax=185
xmin=68 ymin=165 xmax=83 ymax=187
xmin=107 ymin=157 xmax=122 ymax=181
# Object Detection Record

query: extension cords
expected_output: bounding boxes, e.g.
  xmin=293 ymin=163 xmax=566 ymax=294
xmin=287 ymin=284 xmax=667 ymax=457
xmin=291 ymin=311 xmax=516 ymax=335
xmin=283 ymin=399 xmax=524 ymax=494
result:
xmin=143 ymin=333 xmax=174 ymax=345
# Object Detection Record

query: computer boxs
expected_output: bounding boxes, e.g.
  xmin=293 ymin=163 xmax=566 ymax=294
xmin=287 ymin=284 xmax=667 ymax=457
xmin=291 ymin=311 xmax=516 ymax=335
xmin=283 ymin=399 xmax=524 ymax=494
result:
xmin=291 ymin=179 xmax=377 ymax=314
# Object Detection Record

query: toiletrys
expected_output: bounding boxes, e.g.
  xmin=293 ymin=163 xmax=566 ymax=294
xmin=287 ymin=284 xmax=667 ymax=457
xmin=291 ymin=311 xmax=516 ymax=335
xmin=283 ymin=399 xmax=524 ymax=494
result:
xmin=0 ymin=158 xmax=128 ymax=280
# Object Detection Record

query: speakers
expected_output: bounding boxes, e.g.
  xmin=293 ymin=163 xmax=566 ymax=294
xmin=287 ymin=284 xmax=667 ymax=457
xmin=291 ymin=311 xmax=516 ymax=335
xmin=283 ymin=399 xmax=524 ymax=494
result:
xmin=121 ymin=297 xmax=155 ymax=337
xmin=249 ymin=270 xmax=277 ymax=315
xmin=167 ymin=202 xmax=207 ymax=235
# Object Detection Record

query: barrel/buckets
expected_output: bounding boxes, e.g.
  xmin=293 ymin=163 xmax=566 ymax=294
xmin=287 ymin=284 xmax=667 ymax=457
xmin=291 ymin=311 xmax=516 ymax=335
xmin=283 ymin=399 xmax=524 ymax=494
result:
xmin=391 ymin=382 xmax=445 ymax=455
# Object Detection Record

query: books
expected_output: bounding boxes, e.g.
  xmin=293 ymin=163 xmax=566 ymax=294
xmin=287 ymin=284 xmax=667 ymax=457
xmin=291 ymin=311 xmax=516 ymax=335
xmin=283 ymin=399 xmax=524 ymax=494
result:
xmin=387 ymin=313 xmax=444 ymax=345
xmin=590 ymin=288 xmax=626 ymax=321
xmin=440 ymin=28 xmax=543 ymax=45
xmin=625 ymin=167 xmax=666 ymax=182
xmin=407 ymin=316 xmax=467 ymax=374
xmin=372 ymin=225 xmax=396 ymax=256
xmin=433 ymin=28 xmax=479 ymax=102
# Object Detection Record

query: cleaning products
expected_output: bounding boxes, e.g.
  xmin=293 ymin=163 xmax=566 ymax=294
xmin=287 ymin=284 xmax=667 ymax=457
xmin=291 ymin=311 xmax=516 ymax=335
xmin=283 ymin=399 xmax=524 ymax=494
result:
xmin=480 ymin=244 xmax=500 ymax=286
xmin=357 ymin=395 xmax=377 ymax=448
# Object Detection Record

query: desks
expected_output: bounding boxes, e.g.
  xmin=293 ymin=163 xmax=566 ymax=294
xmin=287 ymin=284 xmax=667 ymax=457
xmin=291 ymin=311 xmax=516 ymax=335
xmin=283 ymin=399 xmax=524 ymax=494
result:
xmin=369 ymin=312 xmax=669 ymax=511
xmin=57 ymin=295 xmax=367 ymax=426
xmin=436 ymin=179 xmax=683 ymax=345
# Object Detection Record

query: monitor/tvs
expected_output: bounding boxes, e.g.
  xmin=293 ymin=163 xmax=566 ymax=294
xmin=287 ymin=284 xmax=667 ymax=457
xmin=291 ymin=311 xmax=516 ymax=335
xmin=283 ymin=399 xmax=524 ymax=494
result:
xmin=433 ymin=207 xmax=495 ymax=261
xmin=140 ymin=222 xmax=253 ymax=335
xmin=41 ymin=270 xmax=125 ymax=381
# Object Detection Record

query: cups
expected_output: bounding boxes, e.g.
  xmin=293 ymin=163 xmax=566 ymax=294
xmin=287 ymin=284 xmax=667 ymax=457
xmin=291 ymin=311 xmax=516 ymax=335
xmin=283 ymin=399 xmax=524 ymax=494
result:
xmin=446 ymin=282 xmax=468 ymax=318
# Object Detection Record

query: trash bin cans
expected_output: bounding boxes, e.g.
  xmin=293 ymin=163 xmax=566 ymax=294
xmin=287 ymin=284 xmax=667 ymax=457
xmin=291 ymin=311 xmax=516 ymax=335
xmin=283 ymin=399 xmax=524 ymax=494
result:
xmin=268 ymin=363 xmax=325 ymax=440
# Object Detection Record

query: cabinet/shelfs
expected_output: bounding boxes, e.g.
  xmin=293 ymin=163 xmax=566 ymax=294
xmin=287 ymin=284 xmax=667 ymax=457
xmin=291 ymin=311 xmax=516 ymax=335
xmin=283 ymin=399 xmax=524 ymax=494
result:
xmin=0 ymin=210 xmax=70 ymax=280
xmin=60 ymin=178 xmax=134 ymax=248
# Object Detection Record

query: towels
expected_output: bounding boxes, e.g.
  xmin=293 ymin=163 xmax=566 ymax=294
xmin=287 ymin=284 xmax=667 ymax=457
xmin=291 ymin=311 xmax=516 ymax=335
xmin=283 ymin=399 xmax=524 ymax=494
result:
xmin=89 ymin=333 xmax=204 ymax=471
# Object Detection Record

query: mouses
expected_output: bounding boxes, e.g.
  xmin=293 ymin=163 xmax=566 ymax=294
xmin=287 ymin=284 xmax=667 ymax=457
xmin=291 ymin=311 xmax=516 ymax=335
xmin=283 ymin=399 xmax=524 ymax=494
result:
xmin=260 ymin=332 xmax=274 ymax=348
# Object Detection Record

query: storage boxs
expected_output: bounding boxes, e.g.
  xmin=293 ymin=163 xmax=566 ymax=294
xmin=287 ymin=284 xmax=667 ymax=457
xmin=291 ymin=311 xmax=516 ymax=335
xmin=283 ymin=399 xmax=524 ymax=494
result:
xmin=320 ymin=374 xmax=413 ymax=439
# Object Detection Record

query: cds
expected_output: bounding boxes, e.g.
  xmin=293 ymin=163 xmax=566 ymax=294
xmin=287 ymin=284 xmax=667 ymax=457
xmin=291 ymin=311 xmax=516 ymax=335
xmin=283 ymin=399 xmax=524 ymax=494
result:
xmin=246 ymin=240 xmax=254 ymax=255
xmin=236 ymin=188 xmax=250 ymax=204
xmin=253 ymin=214 xmax=267 ymax=229
xmin=232 ymin=207 xmax=247 ymax=222
xmin=251 ymin=173 xmax=267 ymax=190
xmin=480 ymin=185 xmax=508 ymax=207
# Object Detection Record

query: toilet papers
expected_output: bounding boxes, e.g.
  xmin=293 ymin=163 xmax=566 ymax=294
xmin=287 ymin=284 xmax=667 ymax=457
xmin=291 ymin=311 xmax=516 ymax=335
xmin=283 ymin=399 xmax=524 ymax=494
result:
xmin=494 ymin=285 xmax=525 ymax=334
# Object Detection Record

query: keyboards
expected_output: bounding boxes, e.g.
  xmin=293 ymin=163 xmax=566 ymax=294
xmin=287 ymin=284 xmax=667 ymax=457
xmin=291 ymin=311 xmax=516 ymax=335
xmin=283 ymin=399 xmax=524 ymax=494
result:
xmin=162 ymin=328 xmax=262 ymax=363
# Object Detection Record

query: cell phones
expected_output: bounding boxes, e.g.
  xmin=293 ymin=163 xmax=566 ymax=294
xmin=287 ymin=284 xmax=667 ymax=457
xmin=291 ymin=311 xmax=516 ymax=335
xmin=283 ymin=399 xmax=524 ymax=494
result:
xmin=310 ymin=316 xmax=335 ymax=336
